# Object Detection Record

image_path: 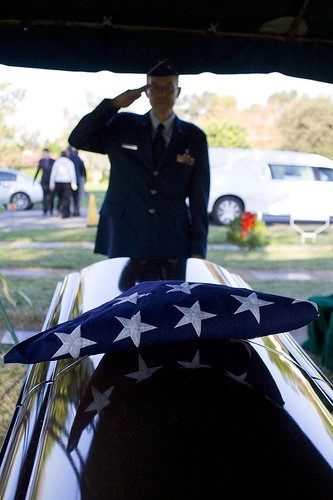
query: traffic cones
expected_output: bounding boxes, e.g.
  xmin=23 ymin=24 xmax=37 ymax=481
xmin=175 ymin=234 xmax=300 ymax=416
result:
xmin=87 ymin=194 xmax=101 ymax=227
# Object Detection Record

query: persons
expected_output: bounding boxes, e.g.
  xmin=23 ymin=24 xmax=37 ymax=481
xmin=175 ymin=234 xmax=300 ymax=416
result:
xmin=32 ymin=146 xmax=87 ymax=219
xmin=67 ymin=58 xmax=211 ymax=290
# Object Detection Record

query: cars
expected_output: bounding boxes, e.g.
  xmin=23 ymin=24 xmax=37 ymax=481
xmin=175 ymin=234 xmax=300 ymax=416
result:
xmin=206 ymin=151 xmax=333 ymax=226
xmin=0 ymin=169 xmax=44 ymax=212
